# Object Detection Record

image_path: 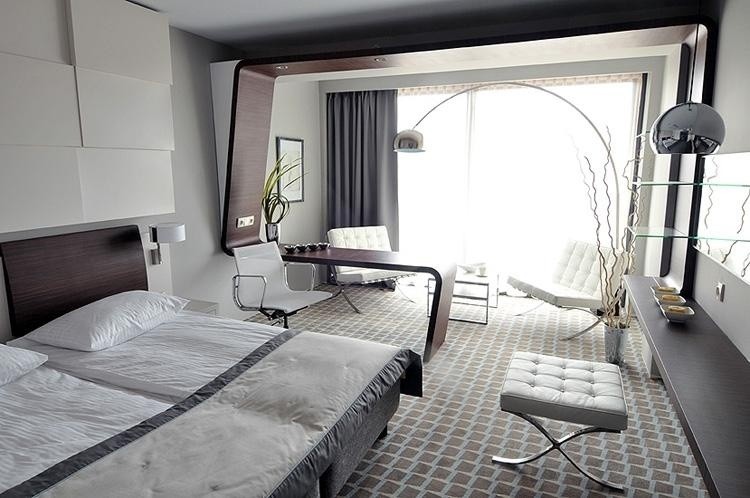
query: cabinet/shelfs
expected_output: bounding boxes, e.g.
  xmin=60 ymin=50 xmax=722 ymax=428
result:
xmin=622 ymin=152 xmax=698 ymax=291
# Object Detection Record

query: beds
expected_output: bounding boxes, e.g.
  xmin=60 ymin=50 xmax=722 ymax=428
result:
xmin=0 ymin=224 xmax=423 ymax=498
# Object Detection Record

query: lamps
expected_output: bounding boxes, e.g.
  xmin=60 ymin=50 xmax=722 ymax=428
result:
xmin=391 ymin=80 xmax=621 ymax=249
xmin=650 ymin=21 xmax=726 ymax=157
xmin=147 ymin=222 xmax=187 ymax=265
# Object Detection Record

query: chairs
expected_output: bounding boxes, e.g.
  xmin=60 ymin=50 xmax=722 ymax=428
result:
xmin=232 ymin=241 xmax=333 ymax=329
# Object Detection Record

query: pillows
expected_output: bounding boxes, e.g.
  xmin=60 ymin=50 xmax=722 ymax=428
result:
xmin=1 ymin=344 xmax=48 ymax=385
xmin=22 ymin=288 xmax=189 ymax=353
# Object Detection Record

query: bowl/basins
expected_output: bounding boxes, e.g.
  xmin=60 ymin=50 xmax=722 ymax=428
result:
xmin=659 ymin=305 xmax=695 ymax=325
xmin=296 ymin=245 xmax=307 ymax=251
xmin=284 ymin=246 xmax=296 ymax=253
xmin=653 ymin=294 xmax=687 ymax=305
xmin=650 ymin=287 xmax=677 ymax=294
xmin=308 ymin=244 xmax=318 ymax=250
xmin=319 ymin=242 xmax=330 ymax=252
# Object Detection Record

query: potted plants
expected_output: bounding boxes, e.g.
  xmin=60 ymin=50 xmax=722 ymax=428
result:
xmin=261 ymin=153 xmax=314 ymax=245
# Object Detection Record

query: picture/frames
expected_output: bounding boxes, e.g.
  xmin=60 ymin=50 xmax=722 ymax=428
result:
xmin=275 ymin=135 xmax=306 ymax=202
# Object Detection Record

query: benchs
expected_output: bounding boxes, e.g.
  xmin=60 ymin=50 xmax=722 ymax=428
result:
xmin=321 ymin=225 xmax=422 ymax=315
xmin=506 ymin=240 xmax=630 ymax=342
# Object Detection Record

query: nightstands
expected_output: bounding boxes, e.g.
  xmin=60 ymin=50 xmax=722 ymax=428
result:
xmin=184 ymin=299 xmax=220 ymax=316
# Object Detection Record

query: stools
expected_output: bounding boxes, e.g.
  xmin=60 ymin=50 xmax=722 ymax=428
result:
xmin=491 ymin=352 xmax=630 ymax=492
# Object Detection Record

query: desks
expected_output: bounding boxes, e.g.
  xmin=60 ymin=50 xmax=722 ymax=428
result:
xmin=227 ymin=238 xmax=458 ymax=362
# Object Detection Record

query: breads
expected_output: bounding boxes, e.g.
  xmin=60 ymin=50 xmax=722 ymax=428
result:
xmin=662 ymin=294 xmax=679 ymax=302
xmin=658 ymin=287 xmax=676 ymax=293
xmin=667 ymin=305 xmax=686 ymax=313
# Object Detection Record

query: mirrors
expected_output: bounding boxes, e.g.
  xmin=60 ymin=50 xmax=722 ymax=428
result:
xmin=687 ymin=151 xmax=750 ymax=285
